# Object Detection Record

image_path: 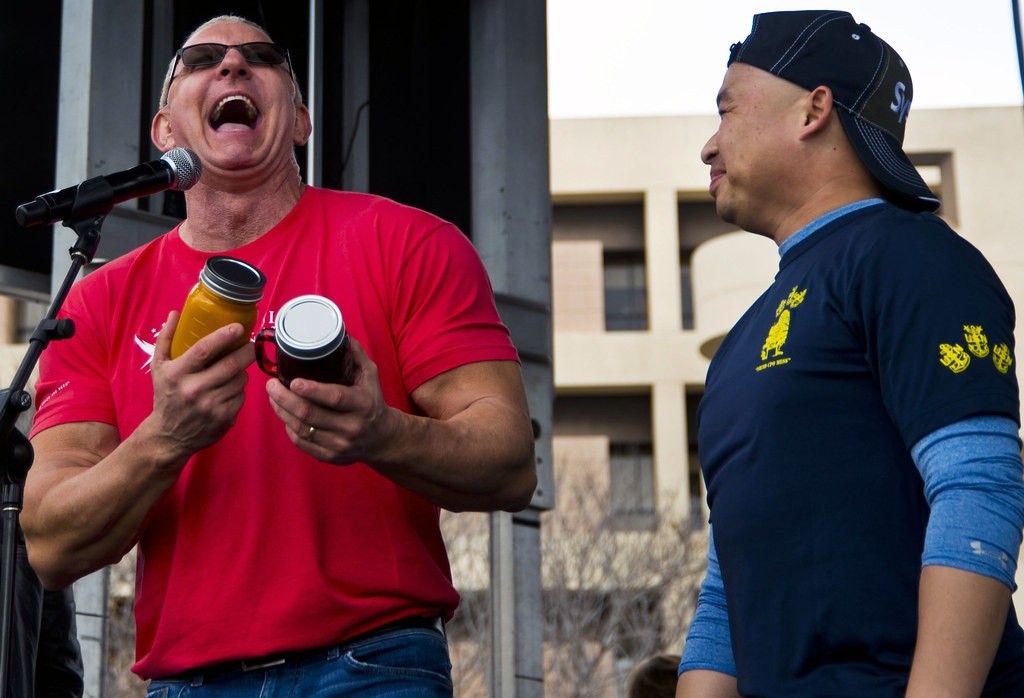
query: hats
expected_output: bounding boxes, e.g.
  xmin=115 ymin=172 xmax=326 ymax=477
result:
xmin=727 ymin=10 xmax=942 ymax=215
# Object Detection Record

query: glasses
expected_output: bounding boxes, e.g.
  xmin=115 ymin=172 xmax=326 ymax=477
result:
xmin=165 ymin=42 xmax=296 ymax=105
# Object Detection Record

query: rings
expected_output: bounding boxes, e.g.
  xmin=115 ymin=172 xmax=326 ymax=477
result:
xmin=308 ymin=426 xmax=317 ymax=441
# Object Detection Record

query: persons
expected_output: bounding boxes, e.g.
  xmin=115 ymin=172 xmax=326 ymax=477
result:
xmin=628 ymin=654 xmax=682 ymax=698
xmin=19 ymin=16 xmax=537 ymax=698
xmin=675 ymin=10 xmax=1024 ymax=698
xmin=0 ymin=386 xmax=85 ymax=698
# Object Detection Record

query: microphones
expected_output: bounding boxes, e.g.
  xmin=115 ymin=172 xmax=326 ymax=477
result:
xmin=14 ymin=148 xmax=203 ymax=229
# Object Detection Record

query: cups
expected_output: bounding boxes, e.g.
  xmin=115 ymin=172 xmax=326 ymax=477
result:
xmin=254 ymin=296 xmax=350 ymax=391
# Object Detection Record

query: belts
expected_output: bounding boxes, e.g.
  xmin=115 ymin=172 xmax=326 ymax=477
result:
xmin=175 ymin=618 xmax=443 ymax=681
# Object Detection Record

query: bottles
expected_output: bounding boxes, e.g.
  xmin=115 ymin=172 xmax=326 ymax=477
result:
xmin=168 ymin=255 xmax=266 ymax=368
